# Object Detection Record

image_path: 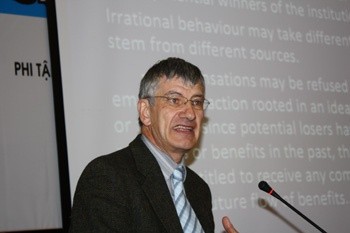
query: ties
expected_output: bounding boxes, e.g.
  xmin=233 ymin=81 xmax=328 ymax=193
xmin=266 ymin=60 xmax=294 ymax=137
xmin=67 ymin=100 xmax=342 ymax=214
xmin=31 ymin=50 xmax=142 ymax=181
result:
xmin=170 ymin=165 xmax=204 ymax=233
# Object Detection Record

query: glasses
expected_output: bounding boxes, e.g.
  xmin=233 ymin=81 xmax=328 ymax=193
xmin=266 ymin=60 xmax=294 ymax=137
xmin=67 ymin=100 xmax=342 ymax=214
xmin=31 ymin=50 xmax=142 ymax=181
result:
xmin=144 ymin=95 xmax=211 ymax=110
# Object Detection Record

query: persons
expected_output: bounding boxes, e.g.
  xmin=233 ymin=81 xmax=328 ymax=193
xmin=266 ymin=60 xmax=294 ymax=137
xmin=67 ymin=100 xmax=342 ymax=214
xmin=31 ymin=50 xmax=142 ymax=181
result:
xmin=68 ymin=57 xmax=241 ymax=233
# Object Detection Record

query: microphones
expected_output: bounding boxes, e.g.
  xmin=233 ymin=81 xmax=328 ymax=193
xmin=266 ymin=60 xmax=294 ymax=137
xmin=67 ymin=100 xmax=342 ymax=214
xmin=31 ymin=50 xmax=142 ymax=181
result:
xmin=258 ymin=181 xmax=327 ymax=233
